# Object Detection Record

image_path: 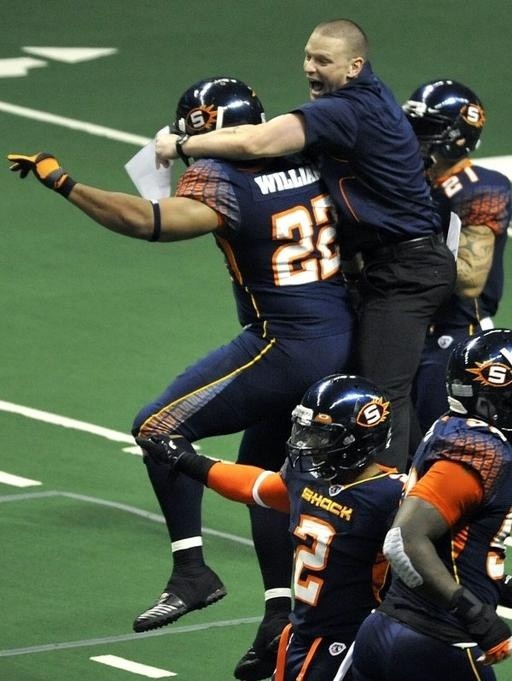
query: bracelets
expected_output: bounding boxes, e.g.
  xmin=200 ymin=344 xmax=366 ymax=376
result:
xmin=175 ymin=133 xmax=194 ymax=160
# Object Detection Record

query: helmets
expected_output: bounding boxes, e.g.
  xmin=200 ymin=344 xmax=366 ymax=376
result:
xmin=175 ymin=77 xmax=266 ymax=167
xmin=402 ymin=80 xmax=485 ymax=175
xmin=444 ymin=329 xmax=511 ymax=435
xmin=284 ymin=373 xmax=394 ymax=482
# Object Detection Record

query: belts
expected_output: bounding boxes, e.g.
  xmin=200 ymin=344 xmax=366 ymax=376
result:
xmin=356 ymin=235 xmax=444 ymax=260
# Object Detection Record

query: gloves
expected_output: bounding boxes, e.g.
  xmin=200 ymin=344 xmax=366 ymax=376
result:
xmin=7 ymin=153 xmax=76 ymax=199
xmin=138 ymin=434 xmax=219 ymax=487
xmin=450 ymin=591 xmax=511 ymax=664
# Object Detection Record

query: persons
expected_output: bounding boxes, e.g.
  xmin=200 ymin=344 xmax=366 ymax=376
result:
xmin=353 ymin=329 xmax=512 ymax=674
xmin=5 ymin=75 xmax=349 ymax=679
xmin=155 ymin=19 xmax=460 ymax=478
xmin=401 ymin=77 xmax=512 ymax=466
xmin=134 ymin=373 xmax=411 ymax=679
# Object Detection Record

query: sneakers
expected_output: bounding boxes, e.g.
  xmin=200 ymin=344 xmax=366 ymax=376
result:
xmin=134 ymin=568 xmax=225 ymax=632
xmin=234 ymin=617 xmax=291 ymax=679
